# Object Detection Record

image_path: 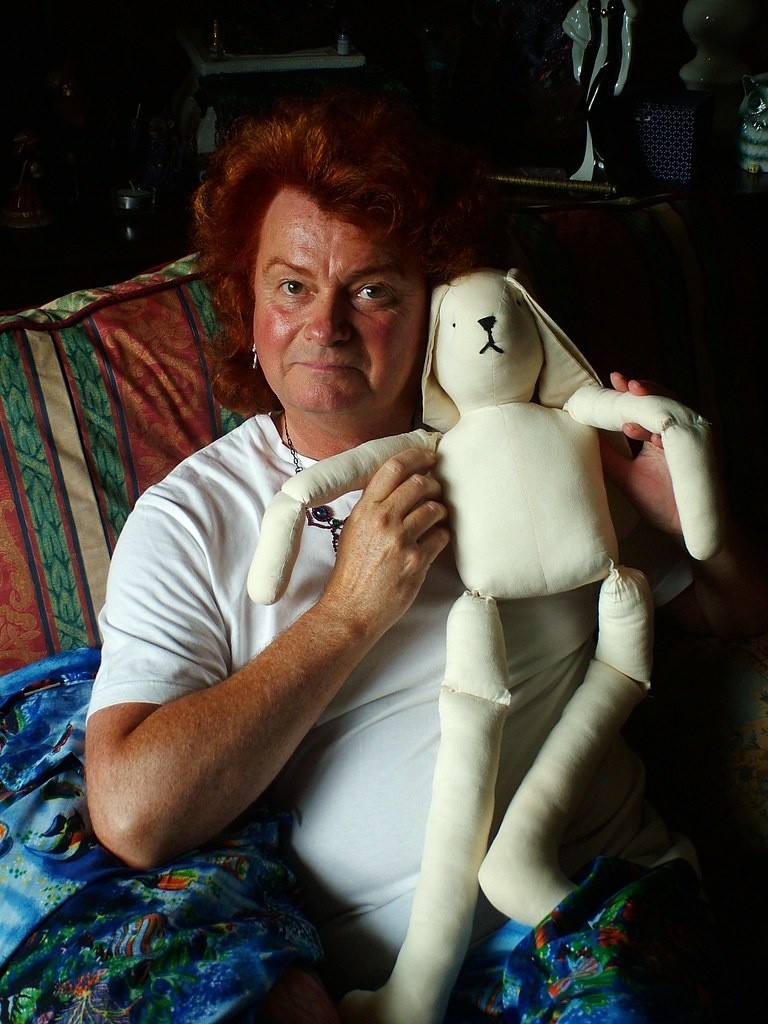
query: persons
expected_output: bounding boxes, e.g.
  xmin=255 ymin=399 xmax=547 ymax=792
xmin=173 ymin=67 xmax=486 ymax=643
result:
xmin=0 ymin=130 xmax=56 ymax=228
xmin=84 ymin=85 xmax=767 ymax=1022
xmin=129 ymin=108 xmax=185 ymax=215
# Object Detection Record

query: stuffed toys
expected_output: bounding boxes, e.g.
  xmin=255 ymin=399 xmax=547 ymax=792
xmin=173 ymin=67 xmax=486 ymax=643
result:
xmin=246 ymin=268 xmax=768 ymax=1024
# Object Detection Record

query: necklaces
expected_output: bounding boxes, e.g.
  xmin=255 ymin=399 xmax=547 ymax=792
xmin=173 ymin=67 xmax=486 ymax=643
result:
xmin=283 ymin=414 xmax=348 ymax=558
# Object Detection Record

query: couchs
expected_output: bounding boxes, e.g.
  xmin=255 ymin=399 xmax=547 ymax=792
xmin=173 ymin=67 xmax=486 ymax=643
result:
xmin=0 ymin=248 xmax=768 ymax=1024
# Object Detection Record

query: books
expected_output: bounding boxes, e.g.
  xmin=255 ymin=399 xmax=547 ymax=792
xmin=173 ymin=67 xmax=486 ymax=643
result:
xmin=177 ymin=28 xmax=365 ymax=75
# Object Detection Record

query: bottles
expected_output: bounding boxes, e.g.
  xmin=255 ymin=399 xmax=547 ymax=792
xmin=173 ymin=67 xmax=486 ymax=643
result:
xmin=208 ymin=20 xmax=225 ymax=60
xmin=336 ymin=22 xmax=352 ymax=55
